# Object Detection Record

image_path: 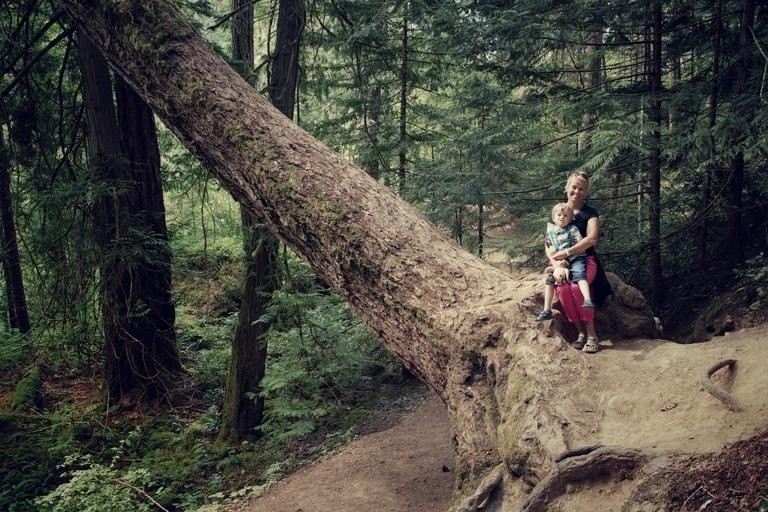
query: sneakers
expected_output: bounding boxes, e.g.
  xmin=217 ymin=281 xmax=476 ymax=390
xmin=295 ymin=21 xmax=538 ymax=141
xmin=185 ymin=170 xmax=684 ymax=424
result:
xmin=572 ymin=333 xmax=601 ymax=353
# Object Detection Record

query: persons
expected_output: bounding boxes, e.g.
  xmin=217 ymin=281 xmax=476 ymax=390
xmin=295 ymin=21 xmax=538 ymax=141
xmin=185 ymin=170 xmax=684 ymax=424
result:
xmin=544 ymin=171 xmax=614 ymax=353
xmin=535 ymin=203 xmax=597 ymax=324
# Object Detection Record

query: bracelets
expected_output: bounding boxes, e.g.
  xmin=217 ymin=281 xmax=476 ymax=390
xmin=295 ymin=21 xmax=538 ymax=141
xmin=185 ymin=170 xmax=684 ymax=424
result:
xmin=565 ymin=249 xmax=572 ymax=257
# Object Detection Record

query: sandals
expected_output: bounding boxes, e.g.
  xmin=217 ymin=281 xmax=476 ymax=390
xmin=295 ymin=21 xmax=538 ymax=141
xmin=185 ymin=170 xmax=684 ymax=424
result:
xmin=533 ymin=310 xmax=554 ymax=320
xmin=580 ymin=298 xmax=596 ymax=309
